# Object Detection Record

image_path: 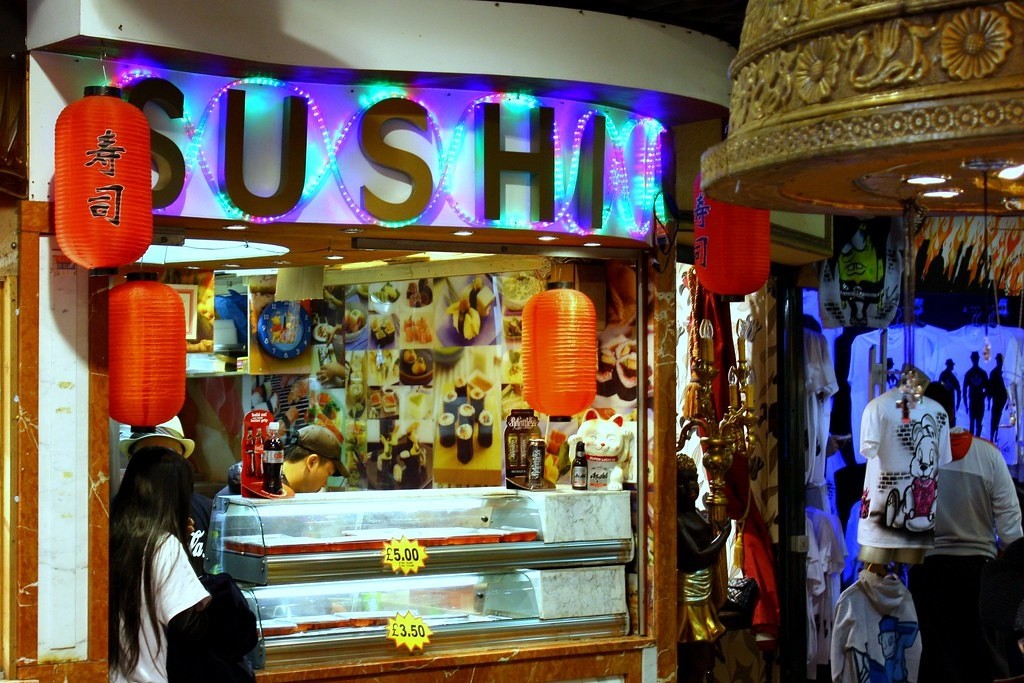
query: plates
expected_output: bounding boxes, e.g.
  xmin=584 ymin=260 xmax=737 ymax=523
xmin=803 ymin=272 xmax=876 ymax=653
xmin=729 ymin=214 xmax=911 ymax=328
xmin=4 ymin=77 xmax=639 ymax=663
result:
xmin=435 ymin=274 xmax=501 ymax=347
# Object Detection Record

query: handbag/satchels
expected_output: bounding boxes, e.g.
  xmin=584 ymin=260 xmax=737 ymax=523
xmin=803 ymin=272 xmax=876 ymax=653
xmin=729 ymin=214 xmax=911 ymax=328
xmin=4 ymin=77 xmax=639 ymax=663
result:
xmin=175 ymin=573 xmax=258 ymax=658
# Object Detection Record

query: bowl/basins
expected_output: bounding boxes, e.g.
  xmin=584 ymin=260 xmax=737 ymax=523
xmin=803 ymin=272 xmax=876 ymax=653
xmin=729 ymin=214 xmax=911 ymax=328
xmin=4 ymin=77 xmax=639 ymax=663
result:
xmin=399 ymin=349 xmax=433 ymax=385
xmin=345 ymin=301 xmax=369 ymax=344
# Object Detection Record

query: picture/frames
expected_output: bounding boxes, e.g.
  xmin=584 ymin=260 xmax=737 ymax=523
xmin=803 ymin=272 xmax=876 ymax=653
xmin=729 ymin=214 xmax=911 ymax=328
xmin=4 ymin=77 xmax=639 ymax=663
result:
xmin=166 ymin=283 xmax=197 ymax=340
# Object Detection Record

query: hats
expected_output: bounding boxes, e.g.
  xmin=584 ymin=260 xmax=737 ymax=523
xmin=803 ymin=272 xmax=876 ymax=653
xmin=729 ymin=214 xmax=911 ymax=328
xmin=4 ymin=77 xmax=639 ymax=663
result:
xmin=118 ymin=415 xmax=195 ymax=459
xmin=296 ymin=424 xmax=351 ymax=478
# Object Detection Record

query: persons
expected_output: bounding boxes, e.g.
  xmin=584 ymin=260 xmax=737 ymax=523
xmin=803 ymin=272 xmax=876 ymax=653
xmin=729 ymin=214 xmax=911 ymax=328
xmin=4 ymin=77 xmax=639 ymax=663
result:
xmin=939 ymin=358 xmax=961 ymax=429
xmin=211 ymin=426 xmax=350 ymax=563
xmin=987 ymin=352 xmax=1008 ymax=440
xmin=924 ymin=381 xmax=1022 ymax=683
xmin=676 ymin=453 xmax=731 ymax=683
xmin=108 ymin=413 xmax=262 ymax=683
xmin=963 ymin=349 xmax=990 ymax=438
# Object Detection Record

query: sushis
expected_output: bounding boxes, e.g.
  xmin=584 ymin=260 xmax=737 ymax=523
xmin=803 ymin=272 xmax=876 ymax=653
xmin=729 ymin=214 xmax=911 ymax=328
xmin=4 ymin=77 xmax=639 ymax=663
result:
xmin=346 ymin=278 xmax=496 ymax=342
xmin=438 ymin=377 xmax=493 ymax=463
xmin=349 ymin=350 xmax=364 ymax=417
xmin=596 ymin=337 xmax=638 ymax=400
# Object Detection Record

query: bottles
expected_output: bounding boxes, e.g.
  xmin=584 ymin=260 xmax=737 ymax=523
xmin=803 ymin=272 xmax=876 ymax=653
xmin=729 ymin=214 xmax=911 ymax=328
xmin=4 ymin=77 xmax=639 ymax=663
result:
xmin=254 ymin=428 xmax=264 ymax=477
xmin=572 ymin=442 xmax=588 ymax=490
xmin=243 ymin=427 xmax=255 ymax=476
xmin=504 ymin=409 xmax=542 ymax=489
xmin=262 ymin=429 xmax=284 ymax=495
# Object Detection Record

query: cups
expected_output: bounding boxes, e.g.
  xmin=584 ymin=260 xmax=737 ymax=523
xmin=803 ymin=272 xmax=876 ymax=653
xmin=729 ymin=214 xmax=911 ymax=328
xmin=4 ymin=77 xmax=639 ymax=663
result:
xmin=382 ymin=386 xmax=395 ymax=395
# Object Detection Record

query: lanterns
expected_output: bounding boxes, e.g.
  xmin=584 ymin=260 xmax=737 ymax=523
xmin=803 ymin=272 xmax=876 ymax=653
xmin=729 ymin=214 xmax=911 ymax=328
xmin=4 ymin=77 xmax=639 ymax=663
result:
xmin=107 ymin=272 xmax=186 ymax=436
xmin=691 ymin=169 xmax=772 ymax=304
xmin=56 ymin=86 xmax=154 ymax=277
xmin=521 ymin=280 xmax=599 ymax=423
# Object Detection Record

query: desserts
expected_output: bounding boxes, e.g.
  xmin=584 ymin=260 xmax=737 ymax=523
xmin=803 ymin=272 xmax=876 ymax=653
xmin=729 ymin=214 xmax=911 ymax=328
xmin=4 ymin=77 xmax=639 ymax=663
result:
xmin=370 ymin=393 xmax=397 ymax=413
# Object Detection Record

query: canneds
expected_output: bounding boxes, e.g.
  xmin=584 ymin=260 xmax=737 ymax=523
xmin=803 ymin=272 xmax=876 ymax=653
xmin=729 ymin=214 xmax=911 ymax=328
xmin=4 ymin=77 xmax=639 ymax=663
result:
xmin=524 ymin=438 xmax=545 ymax=488
